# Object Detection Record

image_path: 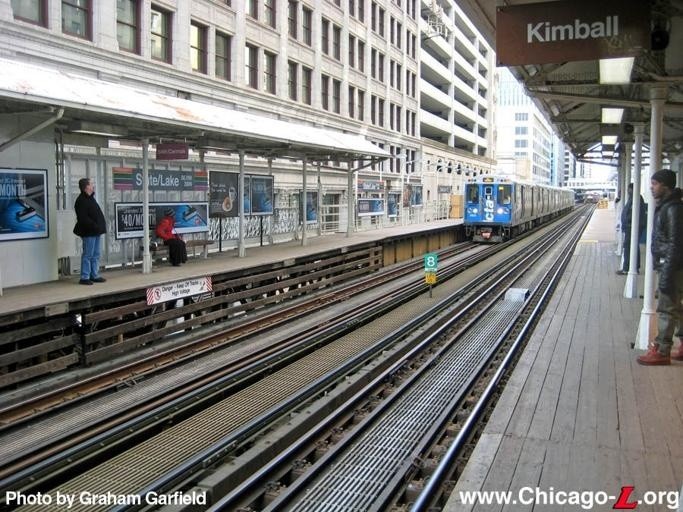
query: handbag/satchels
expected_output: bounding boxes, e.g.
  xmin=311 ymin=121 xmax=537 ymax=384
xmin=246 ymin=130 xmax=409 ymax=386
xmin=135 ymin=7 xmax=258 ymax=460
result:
xmin=151 ymin=230 xmax=164 ymax=245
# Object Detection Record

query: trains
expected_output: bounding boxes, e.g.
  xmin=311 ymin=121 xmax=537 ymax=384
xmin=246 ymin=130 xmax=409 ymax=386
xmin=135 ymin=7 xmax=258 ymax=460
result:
xmin=583 ymin=191 xmax=600 ymax=204
xmin=461 ymin=175 xmax=575 ymax=243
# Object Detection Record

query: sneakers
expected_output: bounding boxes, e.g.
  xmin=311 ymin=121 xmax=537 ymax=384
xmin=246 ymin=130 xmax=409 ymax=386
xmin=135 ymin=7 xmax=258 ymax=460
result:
xmin=617 ymin=268 xmax=640 ymax=274
xmin=671 ymin=336 xmax=683 ymax=360
xmin=79 ymin=277 xmax=106 ymax=285
xmin=637 ymin=343 xmax=669 ymax=365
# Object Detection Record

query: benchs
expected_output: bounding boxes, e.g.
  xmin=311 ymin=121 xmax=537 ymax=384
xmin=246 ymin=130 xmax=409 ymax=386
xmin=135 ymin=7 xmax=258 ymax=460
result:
xmin=139 ymin=240 xmax=215 ymax=269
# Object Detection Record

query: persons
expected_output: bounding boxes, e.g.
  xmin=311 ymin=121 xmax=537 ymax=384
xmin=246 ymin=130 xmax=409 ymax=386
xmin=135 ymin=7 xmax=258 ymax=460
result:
xmin=634 ymin=169 xmax=682 ymax=366
xmin=73 ymin=177 xmax=105 ymax=285
xmin=614 ymin=183 xmax=644 ymax=275
xmin=155 ymin=207 xmax=187 ymax=266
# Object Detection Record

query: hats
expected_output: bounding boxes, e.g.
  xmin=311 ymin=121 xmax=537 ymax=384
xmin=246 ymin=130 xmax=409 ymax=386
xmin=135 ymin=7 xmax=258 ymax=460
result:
xmin=164 ymin=207 xmax=175 ymax=217
xmin=652 ymin=169 xmax=676 ymax=186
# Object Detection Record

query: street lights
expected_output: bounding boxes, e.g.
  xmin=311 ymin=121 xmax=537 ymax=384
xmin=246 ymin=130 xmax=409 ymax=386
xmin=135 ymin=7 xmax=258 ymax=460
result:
xmin=378 ymin=152 xmax=489 ymax=229
